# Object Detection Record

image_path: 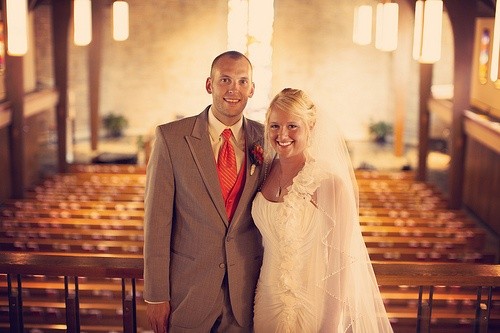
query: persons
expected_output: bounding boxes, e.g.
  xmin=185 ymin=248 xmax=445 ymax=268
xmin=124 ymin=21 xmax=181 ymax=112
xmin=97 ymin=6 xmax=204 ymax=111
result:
xmin=143 ymin=51 xmax=273 ymax=333
xmin=251 ymin=88 xmax=393 ymax=332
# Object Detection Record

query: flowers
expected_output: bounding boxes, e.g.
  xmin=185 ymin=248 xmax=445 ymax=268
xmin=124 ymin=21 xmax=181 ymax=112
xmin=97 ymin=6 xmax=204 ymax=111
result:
xmin=249 ymin=143 xmax=265 ymax=175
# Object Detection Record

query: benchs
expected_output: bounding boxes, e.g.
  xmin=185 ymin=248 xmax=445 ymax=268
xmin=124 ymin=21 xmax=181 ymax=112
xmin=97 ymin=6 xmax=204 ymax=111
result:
xmin=0 ymin=164 xmax=500 ymax=333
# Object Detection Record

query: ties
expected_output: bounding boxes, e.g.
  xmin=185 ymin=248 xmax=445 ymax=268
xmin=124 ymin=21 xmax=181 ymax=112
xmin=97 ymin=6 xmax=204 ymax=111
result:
xmin=217 ymin=128 xmax=238 ymax=209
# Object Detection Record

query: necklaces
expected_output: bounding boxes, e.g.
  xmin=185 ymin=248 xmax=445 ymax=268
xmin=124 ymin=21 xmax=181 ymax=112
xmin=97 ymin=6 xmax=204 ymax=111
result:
xmin=276 ymin=159 xmax=306 ymax=198
